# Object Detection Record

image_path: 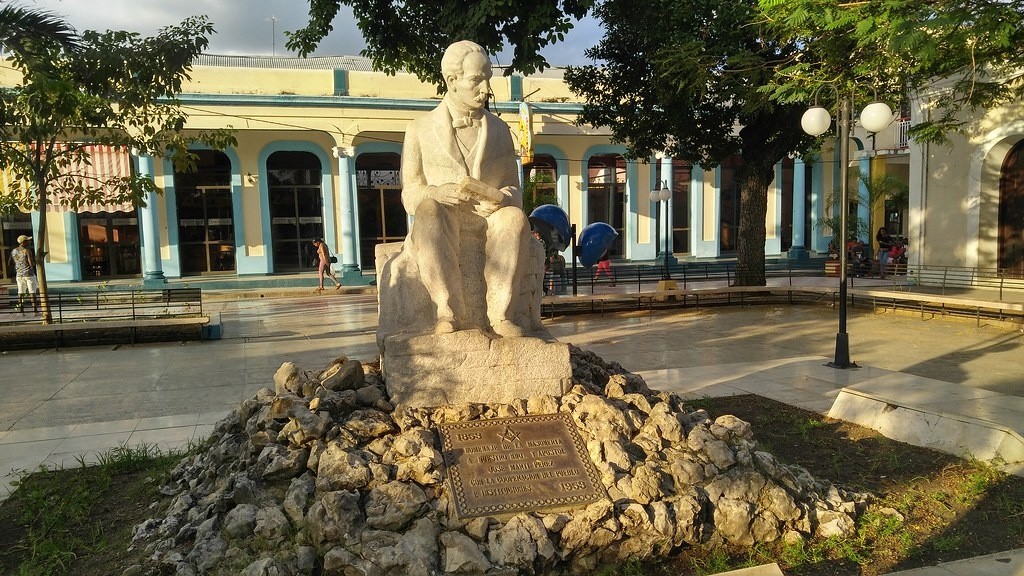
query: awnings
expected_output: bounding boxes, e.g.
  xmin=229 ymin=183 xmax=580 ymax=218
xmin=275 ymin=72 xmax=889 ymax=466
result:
xmin=0 ymin=141 xmax=134 ymax=214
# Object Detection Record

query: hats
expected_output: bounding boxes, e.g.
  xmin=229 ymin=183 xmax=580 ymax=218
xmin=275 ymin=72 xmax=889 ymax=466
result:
xmin=17 ymin=235 xmax=32 ymax=243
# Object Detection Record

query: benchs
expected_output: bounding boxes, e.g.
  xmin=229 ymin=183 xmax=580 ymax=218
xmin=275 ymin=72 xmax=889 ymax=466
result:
xmin=541 ymin=257 xmax=1024 ymax=327
xmin=0 ymin=288 xmax=211 ymax=351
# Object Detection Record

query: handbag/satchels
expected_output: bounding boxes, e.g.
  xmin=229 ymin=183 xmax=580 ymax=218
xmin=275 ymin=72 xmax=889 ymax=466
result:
xmin=329 ymin=257 xmax=337 ymax=263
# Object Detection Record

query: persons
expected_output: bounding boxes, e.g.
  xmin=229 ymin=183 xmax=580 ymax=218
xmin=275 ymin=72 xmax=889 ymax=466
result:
xmin=848 ymin=237 xmax=865 ymax=277
xmin=313 ymin=237 xmax=341 ymax=290
xmin=401 ymin=41 xmax=530 ymax=337
xmin=876 ymin=227 xmax=908 ymax=279
xmin=8 ymin=236 xmax=41 ymax=316
xmin=588 ymin=248 xmax=615 ymax=287
xmin=829 ymin=243 xmax=840 ymax=261
xmin=543 ymin=250 xmax=565 ymax=295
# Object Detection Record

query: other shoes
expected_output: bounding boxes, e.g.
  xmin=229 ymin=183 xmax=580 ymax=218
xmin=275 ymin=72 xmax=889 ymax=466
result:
xmin=34 ymin=313 xmax=42 ymax=316
xmin=315 ymin=287 xmax=324 ymax=290
xmin=608 ymin=283 xmax=615 ymax=287
xmin=335 ymin=284 xmax=341 ymax=290
xmin=880 ymin=276 xmax=885 ymax=280
xmin=16 ymin=313 xmax=24 ymax=316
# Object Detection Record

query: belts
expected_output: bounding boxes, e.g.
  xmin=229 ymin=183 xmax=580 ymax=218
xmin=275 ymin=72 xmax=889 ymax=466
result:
xmin=879 ymin=250 xmax=890 ymax=252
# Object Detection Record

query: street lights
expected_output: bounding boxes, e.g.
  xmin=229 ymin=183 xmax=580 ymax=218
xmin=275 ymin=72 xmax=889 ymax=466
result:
xmin=649 ymin=179 xmax=681 ymax=301
xmin=801 ymin=81 xmax=892 ymax=368
